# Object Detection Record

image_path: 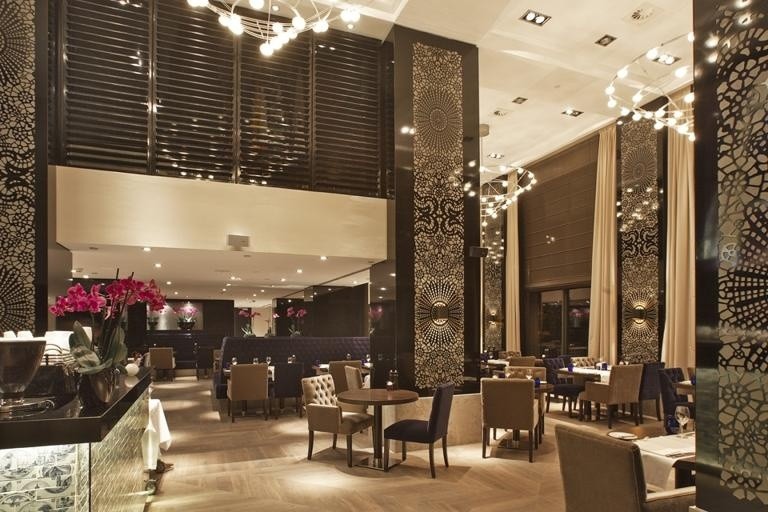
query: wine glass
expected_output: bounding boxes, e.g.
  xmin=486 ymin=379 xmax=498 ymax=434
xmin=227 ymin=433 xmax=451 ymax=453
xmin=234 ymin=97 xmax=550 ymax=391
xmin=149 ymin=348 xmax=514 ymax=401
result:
xmin=624 ymin=357 xmax=629 ymax=365
xmin=232 ymin=357 xmax=237 ymax=366
xmin=541 ymin=353 xmax=546 ymax=362
xmin=674 ymin=406 xmax=690 ymax=439
xmin=572 ymin=360 xmax=577 ymax=370
xmin=504 ymin=368 xmax=511 ymax=378
xmin=266 ymin=356 xmax=272 ymax=365
xmin=488 ymin=352 xmax=494 ymax=361
xmin=346 ymin=353 xmax=351 ymax=361
xmin=366 ymin=353 xmax=371 ymax=361
xmin=525 ymin=369 xmax=533 ymax=380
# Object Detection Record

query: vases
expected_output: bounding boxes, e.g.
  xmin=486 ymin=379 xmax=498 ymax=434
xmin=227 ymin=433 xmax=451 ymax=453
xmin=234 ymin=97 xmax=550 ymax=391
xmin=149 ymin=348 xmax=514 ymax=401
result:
xmin=177 ymin=322 xmax=195 ymax=330
xmin=74 ymin=367 xmax=120 ymax=407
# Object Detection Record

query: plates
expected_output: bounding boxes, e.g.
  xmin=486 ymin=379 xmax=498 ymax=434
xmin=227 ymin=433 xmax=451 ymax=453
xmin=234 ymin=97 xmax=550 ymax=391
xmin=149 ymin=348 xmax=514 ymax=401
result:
xmin=608 ymin=431 xmax=638 ymax=440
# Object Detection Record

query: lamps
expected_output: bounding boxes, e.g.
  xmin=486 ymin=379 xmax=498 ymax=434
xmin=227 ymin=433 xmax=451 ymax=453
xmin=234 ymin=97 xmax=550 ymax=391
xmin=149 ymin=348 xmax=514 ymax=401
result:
xmin=187 ymin=0 xmax=361 ymax=57
xmin=479 ymin=165 xmax=538 ymax=227
xmin=605 ymin=32 xmax=696 ymax=142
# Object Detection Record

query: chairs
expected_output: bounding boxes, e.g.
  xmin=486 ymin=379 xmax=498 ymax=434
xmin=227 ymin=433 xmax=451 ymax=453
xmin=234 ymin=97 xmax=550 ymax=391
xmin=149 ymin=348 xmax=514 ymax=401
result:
xmin=150 ymin=348 xmax=176 ymax=382
xmin=480 ymin=354 xmax=695 ymax=512
xmin=385 ymin=381 xmax=456 ymax=479
xmin=226 ymin=358 xmax=371 ymax=467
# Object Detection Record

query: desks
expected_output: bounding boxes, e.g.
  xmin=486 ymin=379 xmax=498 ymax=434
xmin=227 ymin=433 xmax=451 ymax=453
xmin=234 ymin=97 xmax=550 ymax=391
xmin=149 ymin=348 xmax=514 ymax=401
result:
xmin=338 ymin=389 xmax=419 ymax=471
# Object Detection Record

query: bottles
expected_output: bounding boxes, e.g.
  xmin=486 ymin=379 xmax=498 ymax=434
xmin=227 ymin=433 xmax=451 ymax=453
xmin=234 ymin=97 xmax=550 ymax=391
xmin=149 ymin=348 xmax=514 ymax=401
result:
xmin=393 ymin=369 xmax=400 ymax=391
xmin=292 ymin=355 xmax=297 ymax=364
xmin=388 ymin=369 xmax=394 ymax=381
xmin=362 ymin=355 xmax=366 ymax=365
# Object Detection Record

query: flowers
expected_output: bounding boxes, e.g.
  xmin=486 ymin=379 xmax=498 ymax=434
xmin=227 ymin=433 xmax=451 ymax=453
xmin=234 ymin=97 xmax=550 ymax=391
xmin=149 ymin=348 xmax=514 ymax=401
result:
xmin=238 ymin=310 xmax=260 ymax=338
xmin=178 ymin=301 xmax=198 ymax=322
xmin=49 ymin=268 xmax=166 ymax=375
xmin=287 ymin=307 xmax=307 ymax=336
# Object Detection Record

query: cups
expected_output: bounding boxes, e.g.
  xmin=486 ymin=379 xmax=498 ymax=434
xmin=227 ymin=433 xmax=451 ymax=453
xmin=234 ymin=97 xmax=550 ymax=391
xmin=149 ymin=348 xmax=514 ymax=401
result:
xmin=316 ymin=360 xmax=320 ymax=367
xmin=387 ymin=381 xmax=393 ymax=392
xmin=378 ymin=351 xmax=383 ymax=360
xmin=287 ymin=356 xmax=293 ymax=364
xmin=568 ymin=363 xmax=572 ymax=373
xmin=253 ymin=357 xmax=258 ymax=364
